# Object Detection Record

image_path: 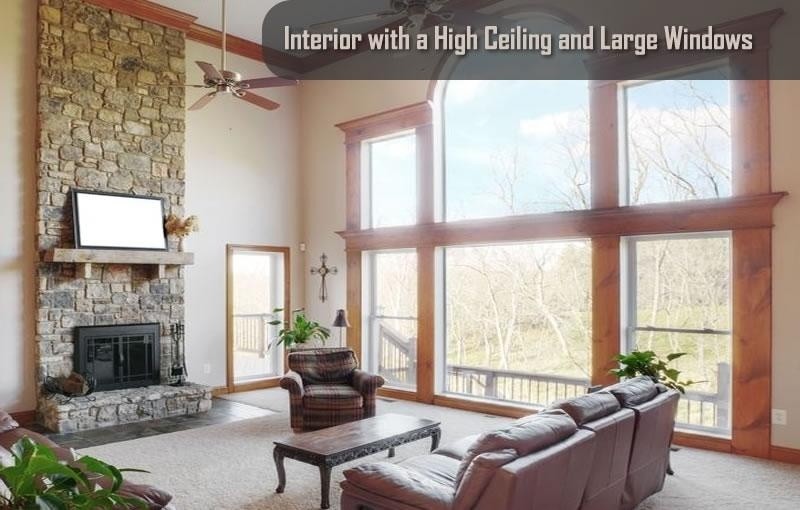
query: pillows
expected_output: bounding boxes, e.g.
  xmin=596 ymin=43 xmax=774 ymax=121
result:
xmin=454 ymin=375 xmax=658 ymax=494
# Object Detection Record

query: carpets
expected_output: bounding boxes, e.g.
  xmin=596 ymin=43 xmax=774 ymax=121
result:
xmin=71 ymin=385 xmax=799 ymax=510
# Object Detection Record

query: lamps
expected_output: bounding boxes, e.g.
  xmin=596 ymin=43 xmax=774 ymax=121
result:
xmin=333 ymin=309 xmax=352 ymax=348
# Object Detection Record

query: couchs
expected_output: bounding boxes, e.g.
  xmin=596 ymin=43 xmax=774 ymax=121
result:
xmin=0 ymin=409 xmax=173 ymax=510
xmin=339 ymin=383 xmax=681 ymax=509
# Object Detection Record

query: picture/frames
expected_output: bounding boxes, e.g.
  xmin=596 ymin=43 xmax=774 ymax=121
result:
xmin=70 ymin=187 xmax=171 ymax=252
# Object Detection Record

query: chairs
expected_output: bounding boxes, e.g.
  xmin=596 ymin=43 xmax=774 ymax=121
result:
xmin=278 ymin=347 xmax=385 ymax=434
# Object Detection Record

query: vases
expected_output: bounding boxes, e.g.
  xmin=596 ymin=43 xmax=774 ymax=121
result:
xmin=177 ymin=238 xmax=184 ymax=251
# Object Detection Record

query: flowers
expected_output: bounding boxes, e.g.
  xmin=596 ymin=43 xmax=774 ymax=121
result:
xmin=164 ymin=213 xmax=201 ymax=239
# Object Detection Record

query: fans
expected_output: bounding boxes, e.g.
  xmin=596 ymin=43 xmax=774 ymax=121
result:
xmin=163 ymin=1 xmax=300 ymax=113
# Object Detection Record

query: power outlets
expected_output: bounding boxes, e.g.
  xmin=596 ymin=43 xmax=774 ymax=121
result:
xmin=770 ymin=408 xmax=789 ymax=427
